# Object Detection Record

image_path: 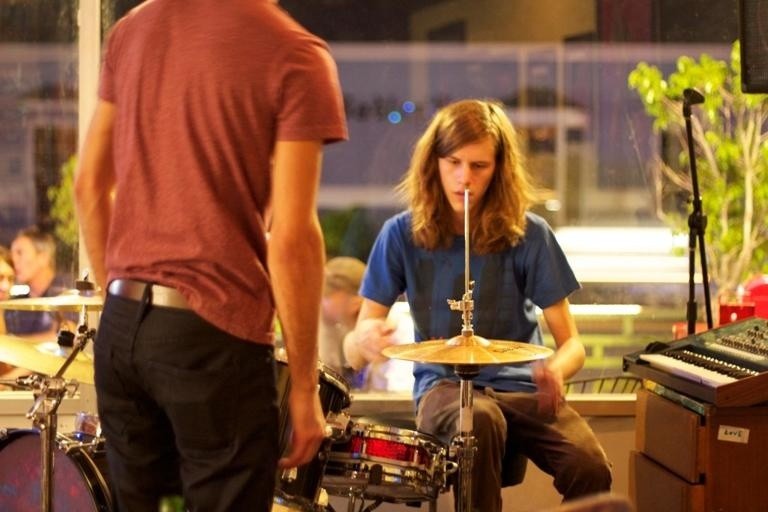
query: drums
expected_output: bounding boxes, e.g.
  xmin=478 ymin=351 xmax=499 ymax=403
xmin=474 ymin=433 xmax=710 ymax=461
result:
xmin=271 ymin=347 xmax=352 ymax=512
xmin=0 ymin=428 xmax=113 ymax=512
xmin=320 ymin=416 xmax=455 ymax=507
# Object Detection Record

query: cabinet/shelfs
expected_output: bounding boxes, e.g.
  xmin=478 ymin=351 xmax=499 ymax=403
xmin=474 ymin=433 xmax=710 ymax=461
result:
xmin=629 ymin=377 xmax=767 ymax=511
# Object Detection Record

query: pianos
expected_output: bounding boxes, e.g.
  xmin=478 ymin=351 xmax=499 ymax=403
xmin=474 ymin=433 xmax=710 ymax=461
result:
xmin=622 ymin=317 xmax=766 ymax=512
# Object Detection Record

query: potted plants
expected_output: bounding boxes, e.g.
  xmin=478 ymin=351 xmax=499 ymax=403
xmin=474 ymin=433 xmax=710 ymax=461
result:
xmin=628 ymin=37 xmax=768 ymax=340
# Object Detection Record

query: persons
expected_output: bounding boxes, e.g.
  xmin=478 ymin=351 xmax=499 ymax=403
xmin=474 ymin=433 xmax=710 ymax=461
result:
xmin=5 ymin=225 xmax=80 ymax=332
xmin=70 ymin=0 xmax=349 ymax=512
xmin=339 ymin=99 xmax=614 ymax=512
xmin=0 ymin=246 xmax=15 ymax=335
xmin=319 ymin=246 xmax=424 ymax=397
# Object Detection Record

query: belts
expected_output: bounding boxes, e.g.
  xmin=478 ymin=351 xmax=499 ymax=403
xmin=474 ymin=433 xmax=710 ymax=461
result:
xmin=108 ymin=278 xmax=192 ymax=311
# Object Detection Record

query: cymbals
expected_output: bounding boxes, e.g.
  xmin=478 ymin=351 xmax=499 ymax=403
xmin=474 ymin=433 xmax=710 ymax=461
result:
xmin=382 ymin=335 xmax=554 ymax=365
xmin=0 ymin=287 xmax=106 ymax=312
xmin=1 ymin=334 xmax=94 ymax=384
